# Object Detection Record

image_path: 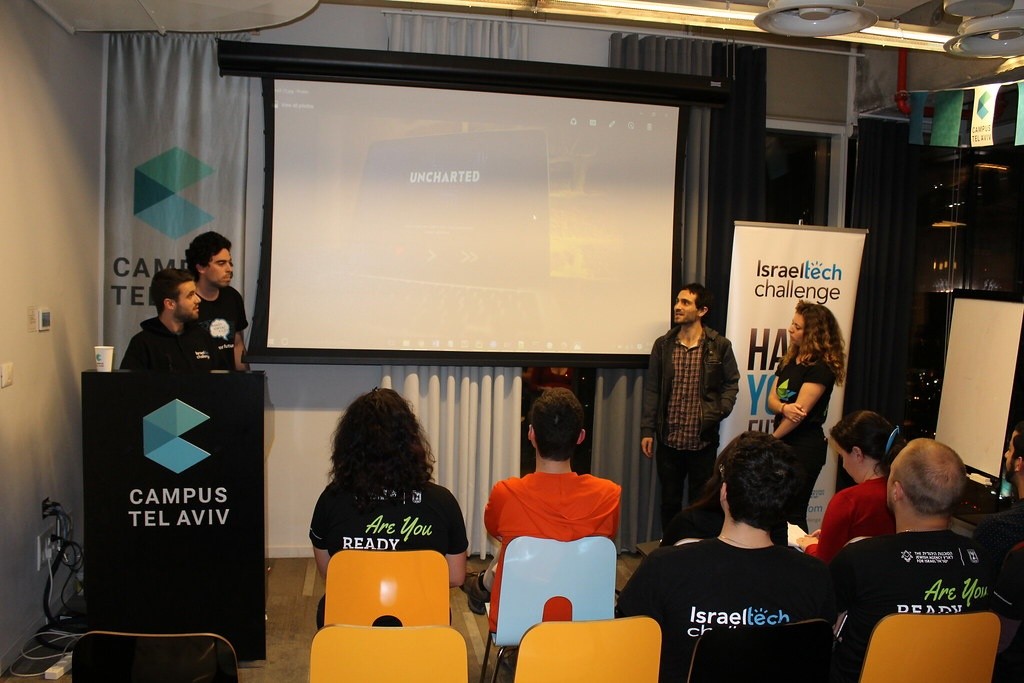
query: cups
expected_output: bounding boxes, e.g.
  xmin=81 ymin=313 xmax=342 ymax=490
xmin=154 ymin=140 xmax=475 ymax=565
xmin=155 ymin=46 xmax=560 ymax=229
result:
xmin=93 ymin=346 xmax=114 ymax=373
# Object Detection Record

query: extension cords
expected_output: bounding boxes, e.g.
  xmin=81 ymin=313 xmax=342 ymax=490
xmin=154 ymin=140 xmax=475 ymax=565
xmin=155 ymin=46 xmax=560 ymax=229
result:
xmin=44 ymin=650 xmax=73 ymax=680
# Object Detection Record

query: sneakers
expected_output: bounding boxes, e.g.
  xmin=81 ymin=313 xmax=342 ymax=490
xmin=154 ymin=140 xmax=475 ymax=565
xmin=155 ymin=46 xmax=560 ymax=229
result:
xmin=457 ymin=567 xmax=490 ymax=615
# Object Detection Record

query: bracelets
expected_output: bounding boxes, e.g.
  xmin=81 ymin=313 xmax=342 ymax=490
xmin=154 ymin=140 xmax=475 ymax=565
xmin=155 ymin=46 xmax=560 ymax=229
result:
xmin=781 ymin=403 xmax=786 ymax=413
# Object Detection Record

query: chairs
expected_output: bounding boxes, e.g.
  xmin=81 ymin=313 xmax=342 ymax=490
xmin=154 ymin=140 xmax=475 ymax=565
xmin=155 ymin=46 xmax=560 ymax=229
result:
xmin=858 ymin=611 xmax=1002 ymax=683
xmin=71 ymin=630 xmax=240 ymax=683
xmin=480 ymin=536 xmax=617 ymax=683
xmin=324 ymin=549 xmax=451 ymax=626
xmin=513 ymin=615 xmax=663 ymax=683
xmin=687 ymin=617 xmax=835 ymax=683
xmin=990 ymin=540 xmax=1024 ymax=683
xmin=308 ymin=623 xmax=469 ymax=683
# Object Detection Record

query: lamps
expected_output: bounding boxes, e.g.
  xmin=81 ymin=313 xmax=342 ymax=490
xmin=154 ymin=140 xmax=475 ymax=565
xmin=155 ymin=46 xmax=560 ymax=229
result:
xmin=408 ymin=0 xmax=536 ymax=13
xmin=536 ymin=0 xmax=961 ymax=52
xmin=753 ymin=0 xmax=879 ymax=38
xmin=942 ymin=0 xmax=1024 ymax=60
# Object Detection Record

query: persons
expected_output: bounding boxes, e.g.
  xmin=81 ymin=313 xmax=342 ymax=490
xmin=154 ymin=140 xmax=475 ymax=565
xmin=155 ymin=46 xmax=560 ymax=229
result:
xmin=461 ymin=387 xmax=621 ymax=614
xmin=799 ymin=411 xmax=906 ymax=563
xmin=120 ymin=268 xmax=228 ymax=369
xmin=830 ymin=438 xmax=994 ymax=683
xmin=615 ymin=431 xmax=837 ymax=683
xmin=660 ymin=435 xmax=741 ymax=547
xmin=188 ymin=231 xmax=251 ymax=370
xmin=310 ymin=388 xmax=468 ymax=629
xmin=973 ymin=421 xmax=1024 ymax=569
xmin=767 ymin=301 xmax=844 ymax=532
xmin=640 ymin=284 xmax=740 ymax=543
xmin=992 ymin=548 xmax=1024 ymax=683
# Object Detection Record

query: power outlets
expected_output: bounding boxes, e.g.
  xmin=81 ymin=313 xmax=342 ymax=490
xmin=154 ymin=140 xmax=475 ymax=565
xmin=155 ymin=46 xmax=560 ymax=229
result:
xmin=58 ymin=511 xmax=74 ymax=551
xmin=41 ymin=497 xmax=50 ymax=521
xmin=36 ymin=524 xmax=58 ymax=572
